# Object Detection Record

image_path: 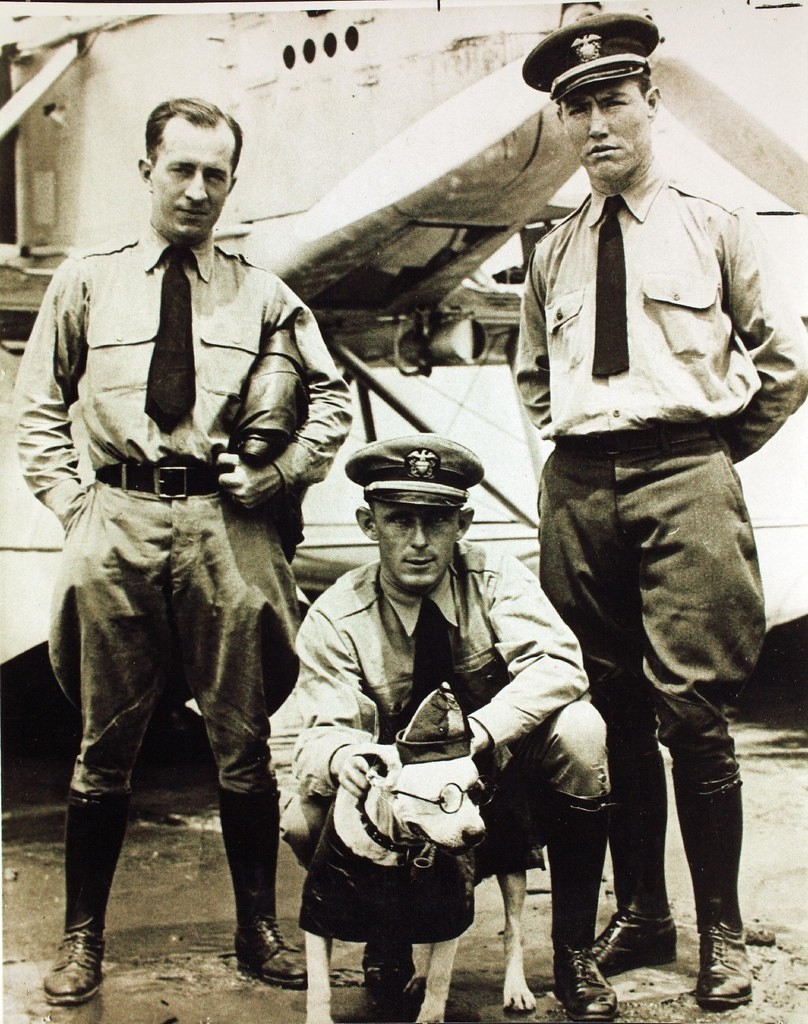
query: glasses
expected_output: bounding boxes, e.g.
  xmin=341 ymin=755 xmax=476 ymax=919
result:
xmin=390 ymin=775 xmax=499 ymax=814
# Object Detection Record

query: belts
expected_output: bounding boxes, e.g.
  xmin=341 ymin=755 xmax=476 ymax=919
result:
xmin=95 ymin=464 xmax=229 ymax=497
xmin=552 ymin=425 xmax=713 ymax=454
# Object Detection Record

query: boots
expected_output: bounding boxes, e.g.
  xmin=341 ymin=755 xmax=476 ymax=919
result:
xmin=550 ymin=784 xmax=618 ymax=1021
xmin=671 ymin=762 xmax=753 ymax=1010
xmin=219 ymin=789 xmax=308 ymax=990
xmin=593 ymin=751 xmax=678 ymax=974
xmin=43 ymin=787 xmax=128 ymax=1004
xmin=361 ymin=940 xmax=416 ymax=991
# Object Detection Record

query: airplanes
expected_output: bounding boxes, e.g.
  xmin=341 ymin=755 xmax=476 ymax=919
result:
xmin=2 ymin=0 xmax=808 ymax=731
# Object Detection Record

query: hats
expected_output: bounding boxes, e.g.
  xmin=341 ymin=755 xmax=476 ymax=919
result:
xmin=521 ymin=13 xmax=659 ymax=102
xmin=344 ymin=433 xmax=484 ymax=507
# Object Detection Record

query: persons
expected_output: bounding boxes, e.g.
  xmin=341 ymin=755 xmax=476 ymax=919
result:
xmin=508 ymin=11 xmax=806 ymax=1014
xmin=276 ymin=433 xmax=622 ymax=1024
xmin=4 ymin=96 xmax=357 ymax=1002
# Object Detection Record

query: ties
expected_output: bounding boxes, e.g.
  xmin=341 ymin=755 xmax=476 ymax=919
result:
xmin=412 ymin=598 xmax=457 ymax=718
xmin=144 ymin=247 xmax=197 ymax=435
xmin=591 ymin=195 xmax=630 ymax=378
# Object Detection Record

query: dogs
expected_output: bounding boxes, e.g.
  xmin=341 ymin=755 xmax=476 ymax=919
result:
xmin=296 ymin=744 xmax=550 ymax=1024
